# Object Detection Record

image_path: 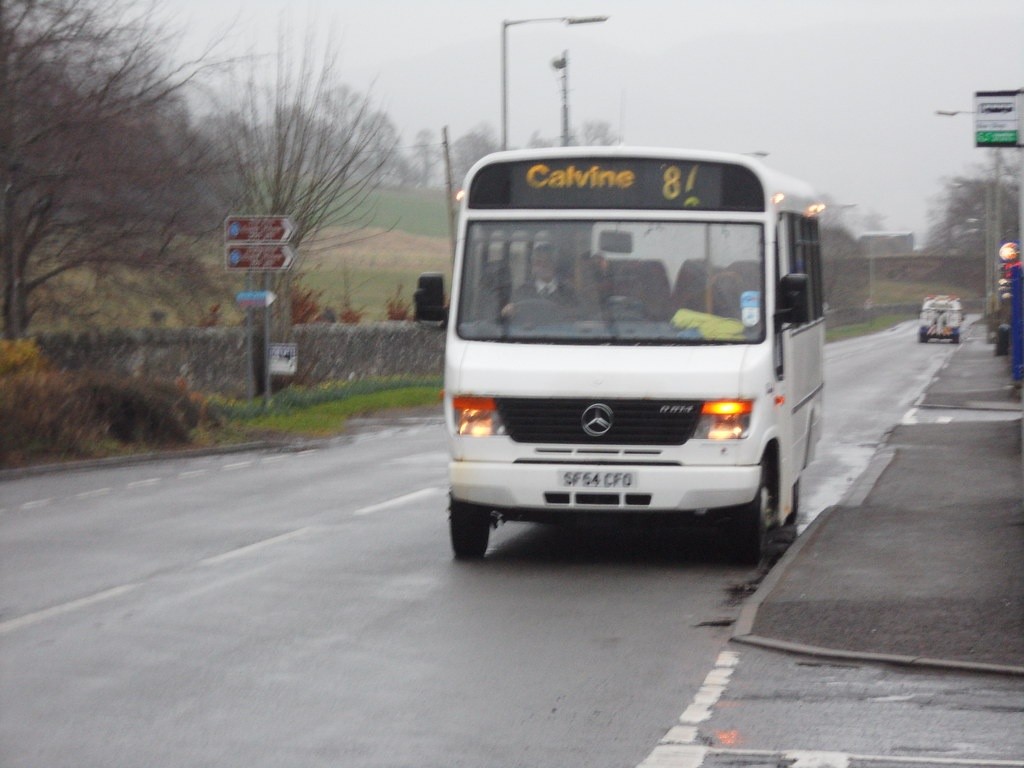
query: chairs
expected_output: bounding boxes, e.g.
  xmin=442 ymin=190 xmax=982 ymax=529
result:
xmin=599 ymin=255 xmax=761 ymax=320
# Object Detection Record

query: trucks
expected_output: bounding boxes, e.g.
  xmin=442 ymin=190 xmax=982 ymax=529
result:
xmin=918 ymin=296 xmax=964 ymax=344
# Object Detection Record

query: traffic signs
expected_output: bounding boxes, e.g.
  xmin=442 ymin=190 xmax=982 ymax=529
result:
xmin=222 ymin=215 xmax=299 ymax=243
xmin=224 ymin=244 xmax=295 ymax=273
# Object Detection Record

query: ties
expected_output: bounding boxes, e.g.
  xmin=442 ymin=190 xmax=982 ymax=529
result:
xmin=541 ymin=286 xmax=550 ymax=302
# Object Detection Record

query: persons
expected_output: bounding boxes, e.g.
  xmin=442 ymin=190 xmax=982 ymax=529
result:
xmin=501 ymin=244 xmax=586 ymax=320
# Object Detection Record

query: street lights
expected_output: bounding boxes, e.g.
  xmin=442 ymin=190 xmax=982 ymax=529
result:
xmin=935 ymin=110 xmax=1001 ymax=318
xmin=552 ymin=48 xmax=570 ymax=149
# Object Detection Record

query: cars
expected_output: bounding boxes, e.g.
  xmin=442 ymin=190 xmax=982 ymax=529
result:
xmin=500 ymin=15 xmax=606 ymax=153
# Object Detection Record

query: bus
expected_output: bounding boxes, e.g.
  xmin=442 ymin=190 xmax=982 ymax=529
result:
xmin=443 ymin=147 xmax=832 ymax=571
